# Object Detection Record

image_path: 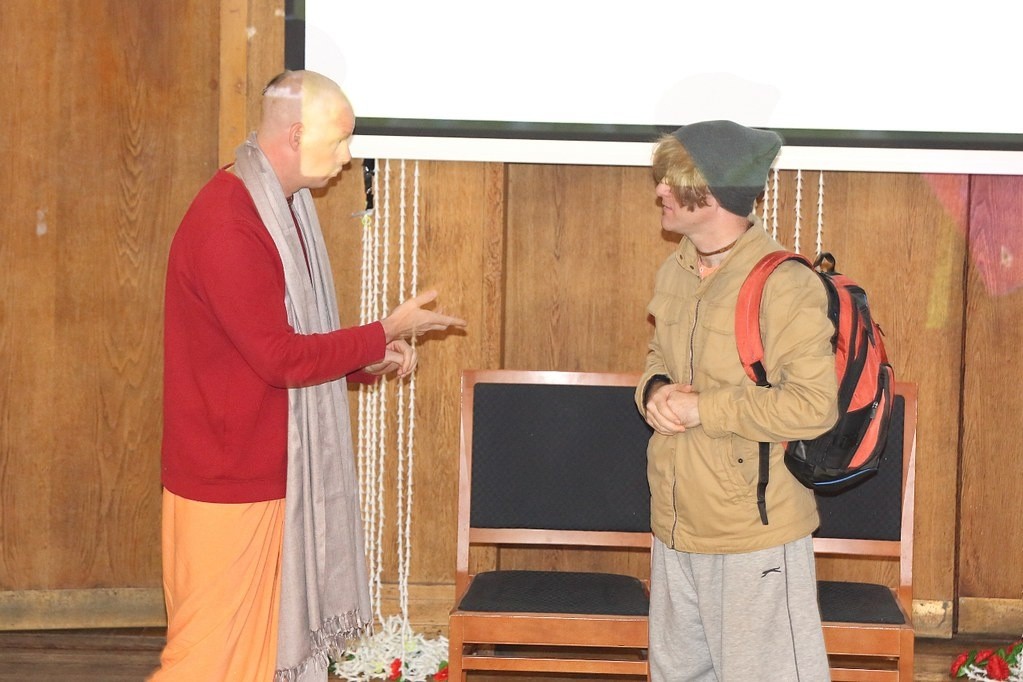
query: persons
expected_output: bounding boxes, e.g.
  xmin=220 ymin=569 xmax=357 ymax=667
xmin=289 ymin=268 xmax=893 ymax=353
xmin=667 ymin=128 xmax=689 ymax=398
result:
xmin=633 ymin=120 xmax=840 ymax=682
xmin=144 ymin=70 xmax=470 ymax=682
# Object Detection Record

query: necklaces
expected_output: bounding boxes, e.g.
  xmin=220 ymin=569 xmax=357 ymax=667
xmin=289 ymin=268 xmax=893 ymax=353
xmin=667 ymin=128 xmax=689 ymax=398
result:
xmin=697 ymin=239 xmax=738 ymax=256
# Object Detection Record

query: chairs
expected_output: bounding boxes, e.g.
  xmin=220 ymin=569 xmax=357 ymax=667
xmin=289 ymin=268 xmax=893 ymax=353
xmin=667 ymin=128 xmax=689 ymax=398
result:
xmin=446 ymin=367 xmax=654 ymax=682
xmin=803 ymin=380 xmax=917 ymax=682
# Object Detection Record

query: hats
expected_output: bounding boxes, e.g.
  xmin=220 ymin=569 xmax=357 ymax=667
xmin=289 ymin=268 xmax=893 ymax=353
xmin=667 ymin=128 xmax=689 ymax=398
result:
xmin=671 ymin=119 xmax=783 ymax=217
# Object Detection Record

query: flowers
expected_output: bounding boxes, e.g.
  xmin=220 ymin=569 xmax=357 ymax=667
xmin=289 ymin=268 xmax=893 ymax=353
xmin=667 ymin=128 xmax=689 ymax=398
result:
xmin=390 ymin=657 xmax=449 ymax=682
xmin=950 ymin=640 xmax=1023 ymax=682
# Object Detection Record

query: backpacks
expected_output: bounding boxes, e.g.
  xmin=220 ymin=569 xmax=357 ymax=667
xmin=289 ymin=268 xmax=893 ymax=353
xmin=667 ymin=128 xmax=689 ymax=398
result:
xmin=734 ymin=249 xmax=896 ymax=495
